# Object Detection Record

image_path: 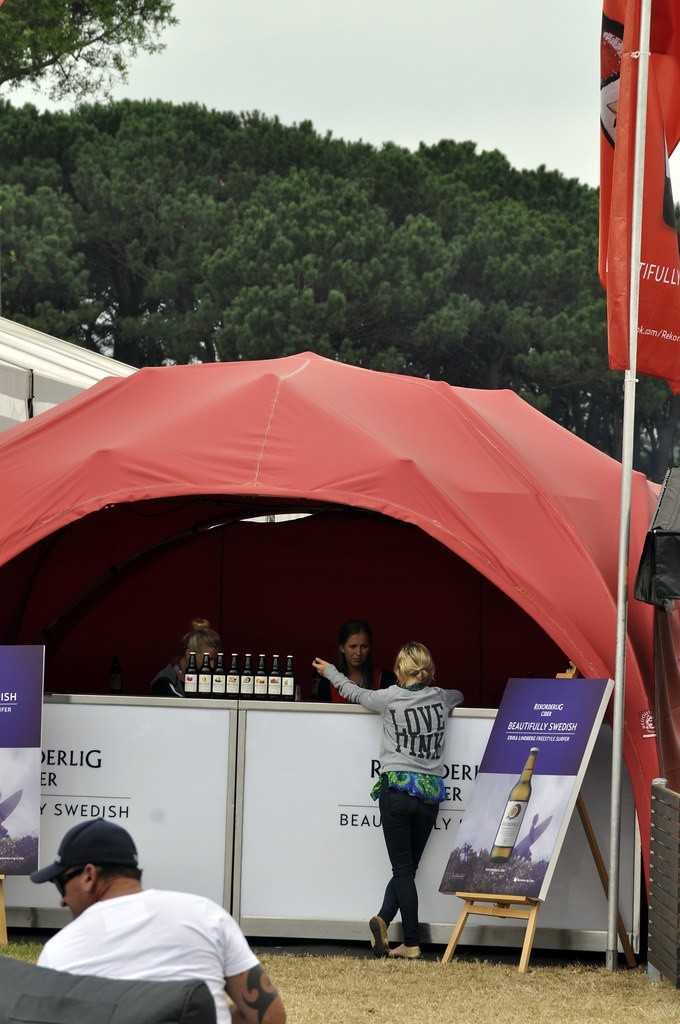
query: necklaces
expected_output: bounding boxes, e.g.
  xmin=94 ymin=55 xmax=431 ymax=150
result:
xmin=176 ymin=660 xmax=185 ymax=673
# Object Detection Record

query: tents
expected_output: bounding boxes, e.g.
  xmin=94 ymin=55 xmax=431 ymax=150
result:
xmin=0 ymin=316 xmax=680 ymax=946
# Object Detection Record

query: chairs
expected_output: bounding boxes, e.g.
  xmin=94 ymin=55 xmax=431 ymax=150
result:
xmin=0 ymin=955 xmax=218 ymax=1024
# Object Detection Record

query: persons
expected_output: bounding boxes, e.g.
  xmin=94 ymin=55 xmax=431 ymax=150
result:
xmin=315 ymin=619 xmax=396 ymax=705
xmin=310 ymin=640 xmax=464 ymax=959
xmin=147 ymin=617 xmax=223 ymax=697
xmin=25 ymin=814 xmax=292 ymax=1024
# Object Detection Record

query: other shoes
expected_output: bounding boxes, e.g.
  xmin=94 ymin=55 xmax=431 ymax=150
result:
xmin=389 ymin=944 xmax=422 ymax=960
xmin=369 ymin=916 xmax=390 ymax=957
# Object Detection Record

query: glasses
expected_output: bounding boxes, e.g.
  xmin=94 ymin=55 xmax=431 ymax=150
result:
xmin=50 ymin=867 xmax=84 ymax=895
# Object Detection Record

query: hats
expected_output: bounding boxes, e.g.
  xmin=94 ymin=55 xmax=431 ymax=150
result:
xmin=29 ymin=817 xmax=139 ymax=884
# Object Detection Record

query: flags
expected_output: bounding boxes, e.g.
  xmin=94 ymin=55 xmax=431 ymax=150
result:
xmin=593 ymin=0 xmax=680 ymax=398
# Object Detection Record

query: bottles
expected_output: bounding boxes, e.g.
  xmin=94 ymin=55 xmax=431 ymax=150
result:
xmin=198 ymin=652 xmax=213 ymax=699
xmin=282 ymin=655 xmax=296 ymax=701
xmin=240 ymin=654 xmax=254 ymax=700
xmin=489 ymin=746 xmax=540 ymax=865
xmin=226 ymin=653 xmax=240 ymax=700
xmin=254 ymin=653 xmax=268 ymax=701
xmin=212 ymin=652 xmax=226 ymax=699
xmin=268 ymin=654 xmax=282 ymax=700
xmin=182 ymin=652 xmax=198 ymax=699
